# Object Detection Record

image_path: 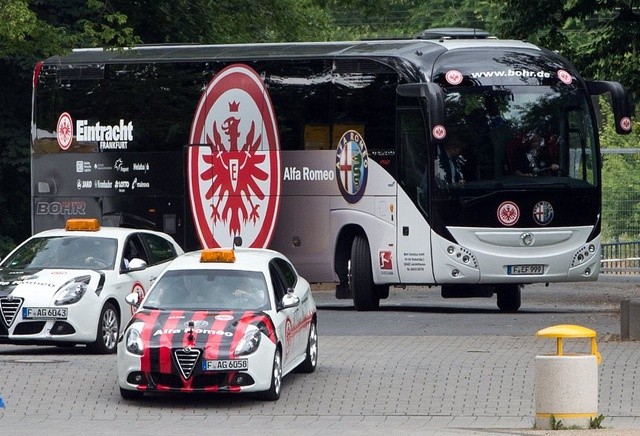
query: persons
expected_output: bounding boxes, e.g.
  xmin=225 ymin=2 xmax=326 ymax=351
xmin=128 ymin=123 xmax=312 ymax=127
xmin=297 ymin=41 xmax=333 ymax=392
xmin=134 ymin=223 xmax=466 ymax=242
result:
xmin=445 ymin=110 xmax=493 ymax=178
xmin=514 ymin=131 xmax=560 ymax=176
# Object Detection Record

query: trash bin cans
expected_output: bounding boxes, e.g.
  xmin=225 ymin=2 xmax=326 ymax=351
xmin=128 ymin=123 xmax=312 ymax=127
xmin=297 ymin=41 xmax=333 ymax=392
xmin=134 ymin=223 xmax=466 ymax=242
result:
xmin=534 ymin=325 xmax=602 ymax=430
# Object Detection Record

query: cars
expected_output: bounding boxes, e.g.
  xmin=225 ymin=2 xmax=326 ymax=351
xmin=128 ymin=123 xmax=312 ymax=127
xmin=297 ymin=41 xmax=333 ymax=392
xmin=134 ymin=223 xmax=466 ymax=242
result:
xmin=0 ymin=218 xmax=187 ymax=354
xmin=117 ymin=247 xmax=318 ymax=400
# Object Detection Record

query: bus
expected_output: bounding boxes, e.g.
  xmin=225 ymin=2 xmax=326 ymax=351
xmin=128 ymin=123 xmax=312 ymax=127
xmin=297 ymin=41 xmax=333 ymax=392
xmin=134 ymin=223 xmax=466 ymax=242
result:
xmin=30 ymin=27 xmax=632 ymax=312
